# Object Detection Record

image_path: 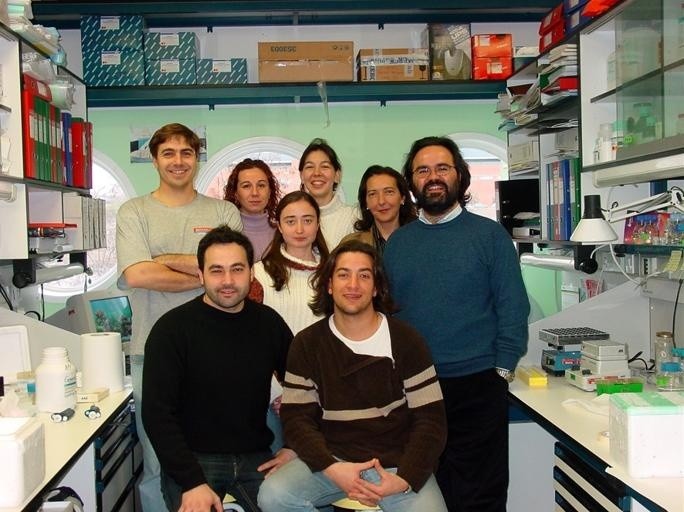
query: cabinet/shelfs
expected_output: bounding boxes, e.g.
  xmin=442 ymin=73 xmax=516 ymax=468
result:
xmin=503 ymin=1 xmax=683 ymax=254
xmin=0 ymin=22 xmax=91 ymax=260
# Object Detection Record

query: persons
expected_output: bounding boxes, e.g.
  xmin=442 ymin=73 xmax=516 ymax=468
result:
xmin=140 ymin=223 xmax=295 ymax=512
xmin=111 ymin=123 xmax=243 ymax=512
xmin=293 ymin=137 xmax=360 ymax=253
xmin=248 ymin=191 xmax=331 ymax=456
xmin=342 ymin=162 xmax=415 ymax=254
xmin=221 ymin=156 xmax=281 ymax=258
xmin=252 ymin=239 xmax=451 ymax=512
xmin=377 ymin=136 xmax=531 ymax=511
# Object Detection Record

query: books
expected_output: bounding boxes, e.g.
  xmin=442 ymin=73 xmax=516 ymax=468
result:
xmin=491 ymin=40 xmax=580 ymax=174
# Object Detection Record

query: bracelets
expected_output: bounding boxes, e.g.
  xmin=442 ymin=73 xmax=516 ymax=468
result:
xmin=405 ymin=484 xmax=412 ymax=496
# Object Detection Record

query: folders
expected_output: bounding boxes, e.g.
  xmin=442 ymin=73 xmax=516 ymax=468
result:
xmin=23 ymin=73 xmax=52 ymax=102
xmin=542 ymin=77 xmax=580 ymax=94
xmin=539 ymin=0 xmax=595 ymax=54
xmin=22 ymin=90 xmax=92 ymax=189
xmin=546 ymin=158 xmax=581 ymax=241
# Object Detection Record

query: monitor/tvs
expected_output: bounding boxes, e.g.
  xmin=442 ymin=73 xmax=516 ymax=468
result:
xmin=42 ymin=290 xmax=137 ymax=361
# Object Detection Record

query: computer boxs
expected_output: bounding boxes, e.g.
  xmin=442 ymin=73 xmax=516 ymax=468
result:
xmin=494 ymin=179 xmax=540 ymax=237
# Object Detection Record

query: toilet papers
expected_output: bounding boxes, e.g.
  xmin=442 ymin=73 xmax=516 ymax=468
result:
xmin=80 ymin=332 xmax=124 ymax=391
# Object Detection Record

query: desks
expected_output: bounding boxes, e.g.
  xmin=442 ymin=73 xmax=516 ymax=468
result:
xmin=498 ymin=358 xmax=683 ymax=512
xmin=2 ymin=384 xmax=136 ymax=512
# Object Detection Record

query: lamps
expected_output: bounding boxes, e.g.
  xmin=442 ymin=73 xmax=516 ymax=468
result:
xmin=566 ymin=187 xmax=684 ymax=244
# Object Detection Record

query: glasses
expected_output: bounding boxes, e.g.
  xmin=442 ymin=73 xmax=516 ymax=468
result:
xmin=416 ymin=163 xmax=454 ymax=178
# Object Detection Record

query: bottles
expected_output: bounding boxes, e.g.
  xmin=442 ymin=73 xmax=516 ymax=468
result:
xmin=654 ymin=332 xmax=675 ymax=371
xmin=34 ymin=349 xmax=75 ymax=411
xmin=632 ymin=213 xmax=683 ymax=246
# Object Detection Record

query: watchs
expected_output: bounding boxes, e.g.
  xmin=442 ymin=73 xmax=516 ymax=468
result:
xmin=495 ymin=365 xmax=516 ymax=383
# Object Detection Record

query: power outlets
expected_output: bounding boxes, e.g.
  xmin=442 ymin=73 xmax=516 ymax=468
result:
xmin=600 ymin=252 xmax=623 ymax=273
